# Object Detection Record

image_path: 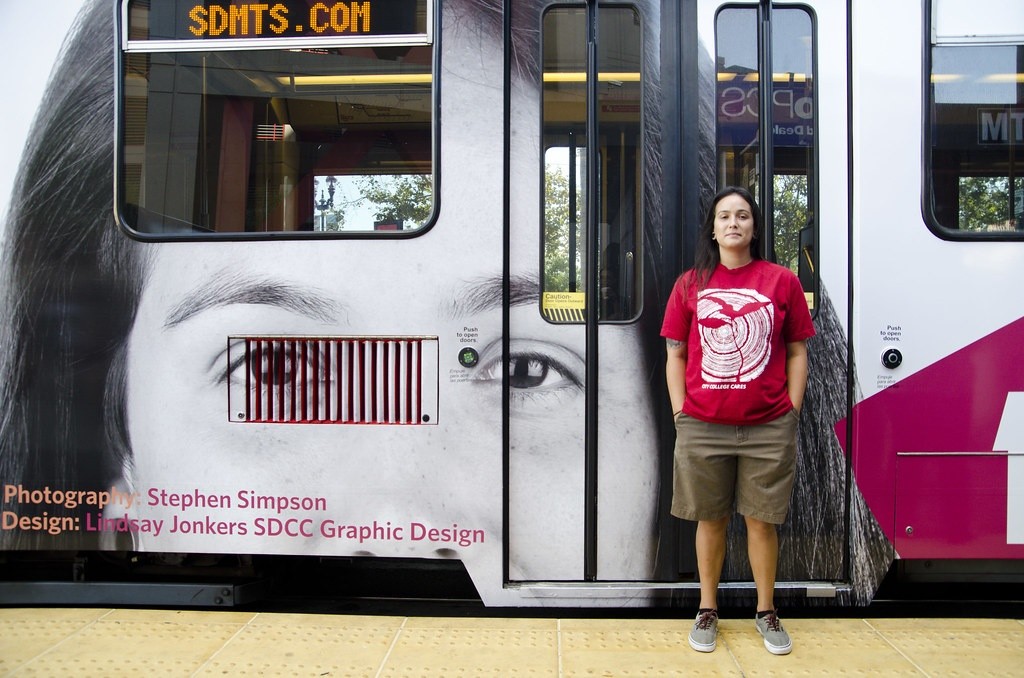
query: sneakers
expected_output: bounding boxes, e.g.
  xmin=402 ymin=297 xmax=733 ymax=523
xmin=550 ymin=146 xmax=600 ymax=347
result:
xmin=689 ymin=605 xmax=718 ymax=653
xmin=755 ymin=608 xmax=792 ymax=655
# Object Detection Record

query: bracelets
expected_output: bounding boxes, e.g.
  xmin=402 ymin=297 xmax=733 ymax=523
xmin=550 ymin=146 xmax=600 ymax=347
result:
xmin=673 ymin=409 xmax=681 ymax=417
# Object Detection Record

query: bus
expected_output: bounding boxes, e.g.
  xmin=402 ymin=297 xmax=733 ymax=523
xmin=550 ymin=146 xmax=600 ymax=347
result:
xmin=0 ymin=2 xmax=1024 ymax=612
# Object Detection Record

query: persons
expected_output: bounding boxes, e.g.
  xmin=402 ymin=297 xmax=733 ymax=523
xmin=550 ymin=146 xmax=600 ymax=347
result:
xmin=659 ymin=186 xmax=816 ymax=657
xmin=2 ymin=5 xmax=900 ymax=613
